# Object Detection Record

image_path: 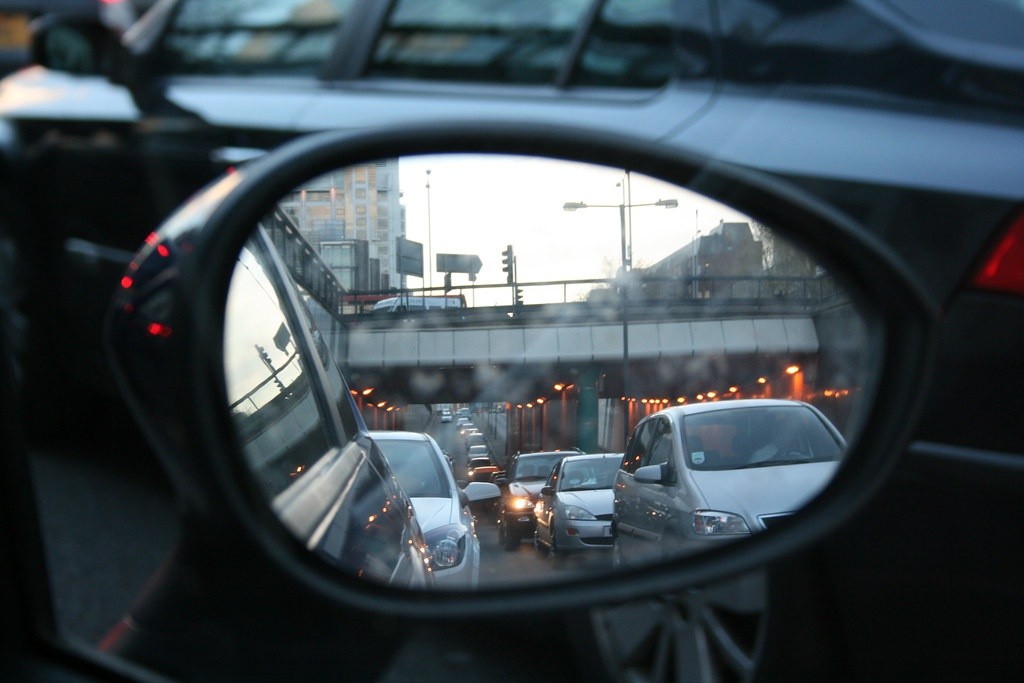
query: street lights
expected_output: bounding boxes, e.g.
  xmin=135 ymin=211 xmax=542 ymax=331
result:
xmin=563 ymin=198 xmax=678 ymax=450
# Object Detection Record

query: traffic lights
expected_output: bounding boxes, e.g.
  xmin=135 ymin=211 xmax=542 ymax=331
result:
xmin=515 ymin=288 xmax=524 ymax=306
xmin=501 ymin=246 xmax=511 ymax=273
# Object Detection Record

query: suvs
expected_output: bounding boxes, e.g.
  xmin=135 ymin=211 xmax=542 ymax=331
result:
xmin=496 ymin=446 xmax=590 ymax=552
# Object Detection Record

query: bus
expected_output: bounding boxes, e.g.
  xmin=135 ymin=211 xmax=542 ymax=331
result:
xmin=371 ymin=297 xmax=463 ymax=313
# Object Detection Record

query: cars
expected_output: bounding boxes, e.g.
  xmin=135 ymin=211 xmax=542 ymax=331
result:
xmin=440 ymin=406 xmax=507 ymax=524
xmin=225 ymin=220 xmax=438 ymax=596
xmin=609 ymin=398 xmax=853 ymax=567
xmin=360 ymin=431 xmax=502 ymax=600
xmin=533 ymin=452 xmax=627 ymax=566
